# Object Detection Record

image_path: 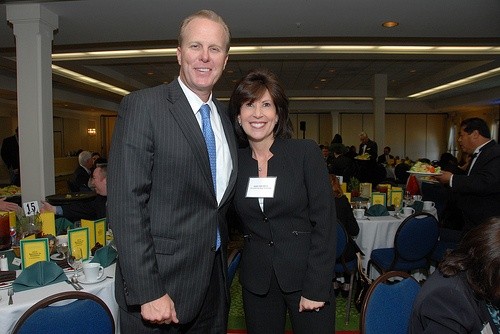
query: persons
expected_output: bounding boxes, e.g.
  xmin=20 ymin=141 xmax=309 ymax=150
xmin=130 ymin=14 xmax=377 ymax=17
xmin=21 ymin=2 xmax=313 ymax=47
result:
xmin=107 ymin=10 xmax=239 ymax=334
xmin=318 ymin=115 xmax=500 ymax=334
xmin=227 ymin=69 xmax=337 ymax=334
xmin=0 ymin=125 xmax=108 ymax=223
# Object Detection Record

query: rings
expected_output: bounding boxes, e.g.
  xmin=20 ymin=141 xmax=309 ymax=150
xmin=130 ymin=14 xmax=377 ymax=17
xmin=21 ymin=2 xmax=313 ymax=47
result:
xmin=315 ymin=308 xmax=319 ymax=311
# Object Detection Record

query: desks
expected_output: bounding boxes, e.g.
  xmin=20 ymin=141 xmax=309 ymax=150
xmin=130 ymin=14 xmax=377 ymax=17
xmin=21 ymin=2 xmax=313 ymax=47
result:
xmin=421 ymin=182 xmax=446 ymax=213
xmin=352 ymin=206 xmax=438 ymax=280
xmin=0 ymin=235 xmax=120 ymax=334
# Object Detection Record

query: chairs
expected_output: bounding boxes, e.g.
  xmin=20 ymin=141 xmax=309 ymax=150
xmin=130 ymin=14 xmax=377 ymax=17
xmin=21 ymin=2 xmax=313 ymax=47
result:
xmin=366 ymin=212 xmax=438 ymax=278
xmin=359 ymin=271 xmax=420 ymax=334
xmin=11 ymin=291 xmax=115 ymax=334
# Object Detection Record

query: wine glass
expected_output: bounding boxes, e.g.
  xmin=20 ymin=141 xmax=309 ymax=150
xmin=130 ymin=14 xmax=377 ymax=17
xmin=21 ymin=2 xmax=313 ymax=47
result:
xmin=67 ymin=247 xmax=83 ymax=277
xmin=393 ymin=197 xmax=403 ymax=217
xmin=404 ymin=191 xmax=415 ymax=207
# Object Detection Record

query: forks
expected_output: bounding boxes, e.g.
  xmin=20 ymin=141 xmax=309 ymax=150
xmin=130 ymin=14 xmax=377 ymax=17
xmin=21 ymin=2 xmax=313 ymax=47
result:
xmin=8 ymin=288 xmax=14 ymax=305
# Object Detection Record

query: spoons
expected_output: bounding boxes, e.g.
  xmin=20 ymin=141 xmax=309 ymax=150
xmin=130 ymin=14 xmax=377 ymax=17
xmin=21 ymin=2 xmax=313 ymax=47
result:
xmin=71 ymin=276 xmax=84 ymax=289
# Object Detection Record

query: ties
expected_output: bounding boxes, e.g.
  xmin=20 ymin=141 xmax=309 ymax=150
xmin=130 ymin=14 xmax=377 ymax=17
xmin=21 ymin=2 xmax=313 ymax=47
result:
xmin=465 ymin=143 xmax=488 ymax=176
xmin=387 ymin=155 xmax=388 ymax=161
xmin=200 ymin=105 xmax=221 ymax=251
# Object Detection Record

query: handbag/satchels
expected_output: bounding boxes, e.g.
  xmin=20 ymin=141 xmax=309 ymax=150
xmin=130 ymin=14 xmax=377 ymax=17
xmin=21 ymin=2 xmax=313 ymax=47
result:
xmin=355 ymin=284 xmax=369 ymax=312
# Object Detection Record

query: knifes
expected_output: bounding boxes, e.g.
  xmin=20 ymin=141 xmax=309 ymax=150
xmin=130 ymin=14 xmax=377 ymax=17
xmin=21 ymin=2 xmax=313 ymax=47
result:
xmin=65 ymin=279 xmax=80 ymax=290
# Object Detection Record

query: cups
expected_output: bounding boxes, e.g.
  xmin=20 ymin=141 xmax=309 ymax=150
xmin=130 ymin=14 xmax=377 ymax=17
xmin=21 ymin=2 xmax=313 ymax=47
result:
xmin=423 ymin=202 xmax=435 ymax=211
xmin=376 ymin=185 xmax=388 ymax=193
xmin=414 ymin=195 xmax=422 ymax=201
xmin=353 ymin=209 xmax=365 ymax=218
xmin=355 ymin=201 xmax=368 ymax=211
xmin=82 ymin=263 xmax=104 ymax=281
xmin=0 ymin=209 xmax=12 ymax=251
xmin=18 ymin=215 xmax=43 ymax=241
xmin=403 ymin=207 xmax=415 ymax=216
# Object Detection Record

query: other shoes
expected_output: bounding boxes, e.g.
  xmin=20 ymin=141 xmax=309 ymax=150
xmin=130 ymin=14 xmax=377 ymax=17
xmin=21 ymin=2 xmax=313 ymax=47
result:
xmin=334 ymin=285 xmax=341 ymax=295
xmin=341 ymin=290 xmax=349 ymax=298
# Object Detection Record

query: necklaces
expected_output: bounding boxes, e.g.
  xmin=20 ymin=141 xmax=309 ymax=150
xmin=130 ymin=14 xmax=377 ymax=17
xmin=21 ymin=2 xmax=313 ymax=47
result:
xmin=257 ymin=153 xmax=271 ymax=171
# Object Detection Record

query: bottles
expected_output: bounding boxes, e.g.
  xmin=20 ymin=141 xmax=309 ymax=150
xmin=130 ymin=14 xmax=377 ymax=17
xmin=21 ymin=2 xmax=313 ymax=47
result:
xmin=8 ymin=225 xmax=17 ymax=249
xmin=0 ymin=254 xmax=9 ymax=271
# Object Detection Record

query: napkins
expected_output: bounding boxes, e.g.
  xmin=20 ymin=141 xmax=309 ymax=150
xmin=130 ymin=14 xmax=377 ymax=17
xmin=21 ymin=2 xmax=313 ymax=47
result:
xmin=12 ymin=261 xmax=67 ymax=292
xmin=56 ymin=217 xmax=74 ymax=235
xmin=88 ymin=244 xmax=117 ymax=268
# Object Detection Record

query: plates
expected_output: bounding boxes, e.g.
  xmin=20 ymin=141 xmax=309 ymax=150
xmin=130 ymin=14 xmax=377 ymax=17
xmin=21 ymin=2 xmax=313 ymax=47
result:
xmin=0 ymin=193 xmax=21 ymax=197
xmin=354 ymin=157 xmax=370 ymax=160
xmin=106 ymin=265 xmax=115 ymax=278
xmin=78 ymin=275 xmax=107 ymax=284
xmin=354 ymin=216 xmax=368 ymax=219
xmin=418 ymin=179 xmax=440 ymax=185
xmin=406 ymin=170 xmax=443 ymax=176
xmin=401 ymin=214 xmax=408 ymax=218
